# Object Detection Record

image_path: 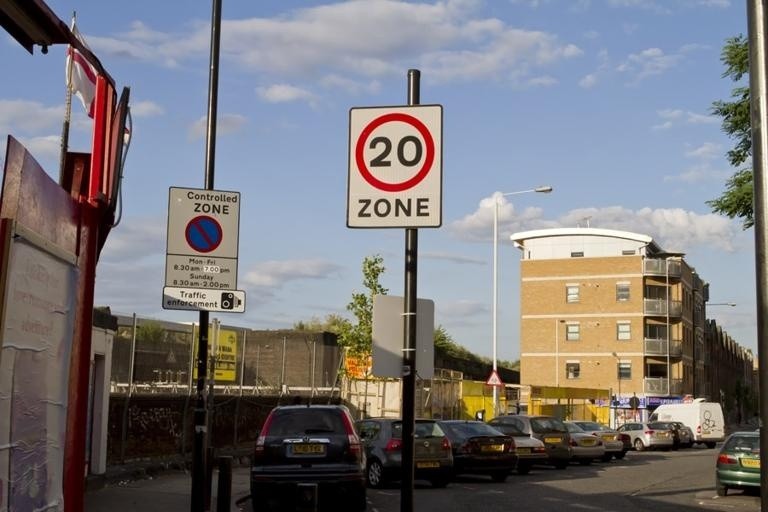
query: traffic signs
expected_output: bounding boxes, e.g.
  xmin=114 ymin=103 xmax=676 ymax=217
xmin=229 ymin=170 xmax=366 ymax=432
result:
xmin=345 ymin=103 xmax=443 ymax=230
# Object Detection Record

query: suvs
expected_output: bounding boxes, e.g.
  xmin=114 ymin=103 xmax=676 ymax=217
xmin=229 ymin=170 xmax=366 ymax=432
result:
xmin=488 ymin=417 xmax=570 ymax=469
xmin=247 ymin=400 xmax=370 ymax=512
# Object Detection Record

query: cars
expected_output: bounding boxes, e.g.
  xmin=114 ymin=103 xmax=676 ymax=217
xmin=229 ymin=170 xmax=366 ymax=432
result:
xmin=442 ymin=420 xmax=516 ymax=484
xmin=715 ymin=430 xmax=760 ymax=497
xmin=487 ymin=422 xmax=548 ymax=476
xmin=649 ymin=420 xmax=695 ymax=448
xmin=612 ymin=433 xmax=633 ymax=459
xmin=561 ymin=422 xmax=605 ymax=467
xmin=616 ymin=422 xmax=675 ymax=452
xmin=577 ymin=421 xmax=624 ymax=463
xmin=353 ymin=418 xmax=455 ymax=490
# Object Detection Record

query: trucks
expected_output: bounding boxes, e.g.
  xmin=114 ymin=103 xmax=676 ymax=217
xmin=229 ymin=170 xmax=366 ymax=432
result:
xmin=643 ymin=395 xmax=727 ymax=451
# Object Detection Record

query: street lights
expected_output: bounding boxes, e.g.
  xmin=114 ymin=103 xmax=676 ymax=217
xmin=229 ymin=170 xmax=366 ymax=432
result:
xmin=553 ymin=318 xmax=566 ymax=386
xmin=254 ymin=343 xmax=269 ymax=387
xmin=665 ymin=254 xmax=683 ymax=397
xmin=492 ymin=182 xmax=553 ymax=420
xmin=690 ymin=301 xmax=739 ymax=398
